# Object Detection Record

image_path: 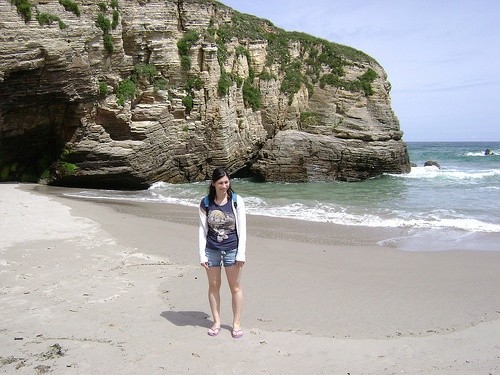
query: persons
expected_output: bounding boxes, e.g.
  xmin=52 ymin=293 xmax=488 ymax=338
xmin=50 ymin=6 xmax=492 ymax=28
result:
xmin=197 ymin=168 xmax=246 ymax=339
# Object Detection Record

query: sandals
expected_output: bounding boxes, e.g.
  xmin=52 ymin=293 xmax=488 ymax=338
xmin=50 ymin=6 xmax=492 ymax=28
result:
xmin=232 ymin=329 xmax=243 ymax=338
xmin=207 ymin=327 xmax=220 ymax=336
